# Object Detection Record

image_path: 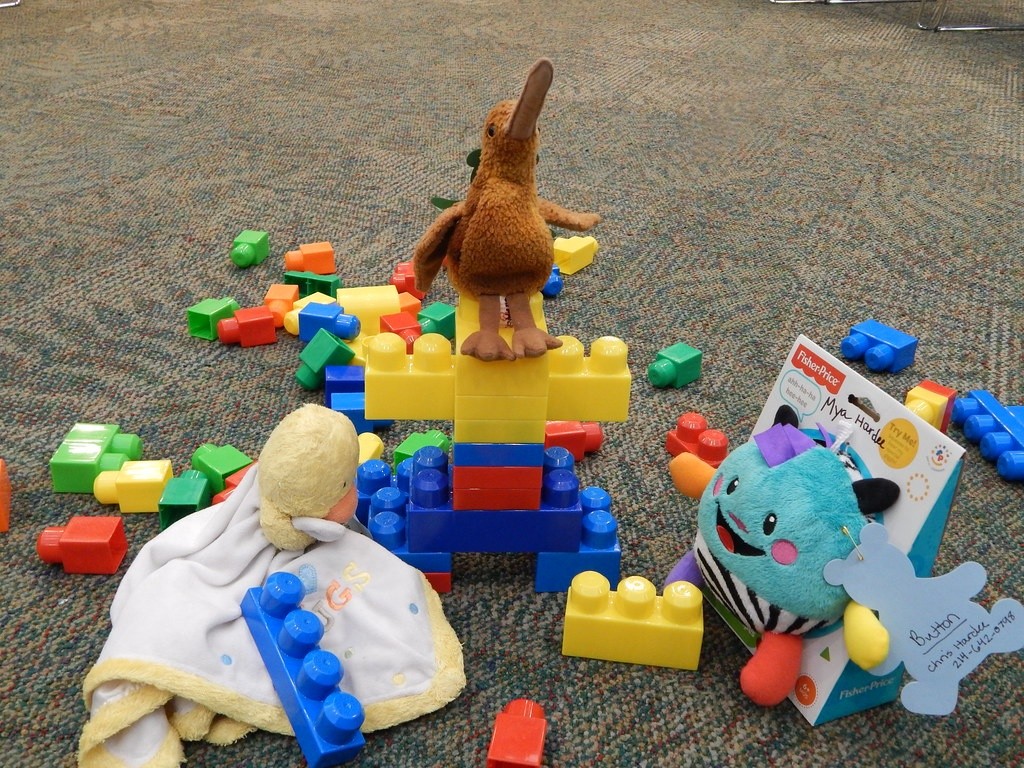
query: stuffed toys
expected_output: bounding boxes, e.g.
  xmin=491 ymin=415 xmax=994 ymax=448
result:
xmin=413 ymin=61 xmax=603 ymax=362
xmin=664 ymin=422 xmax=890 ymax=707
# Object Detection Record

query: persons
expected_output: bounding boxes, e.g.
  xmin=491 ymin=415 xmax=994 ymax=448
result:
xmin=258 ymin=404 xmax=359 ymax=552
